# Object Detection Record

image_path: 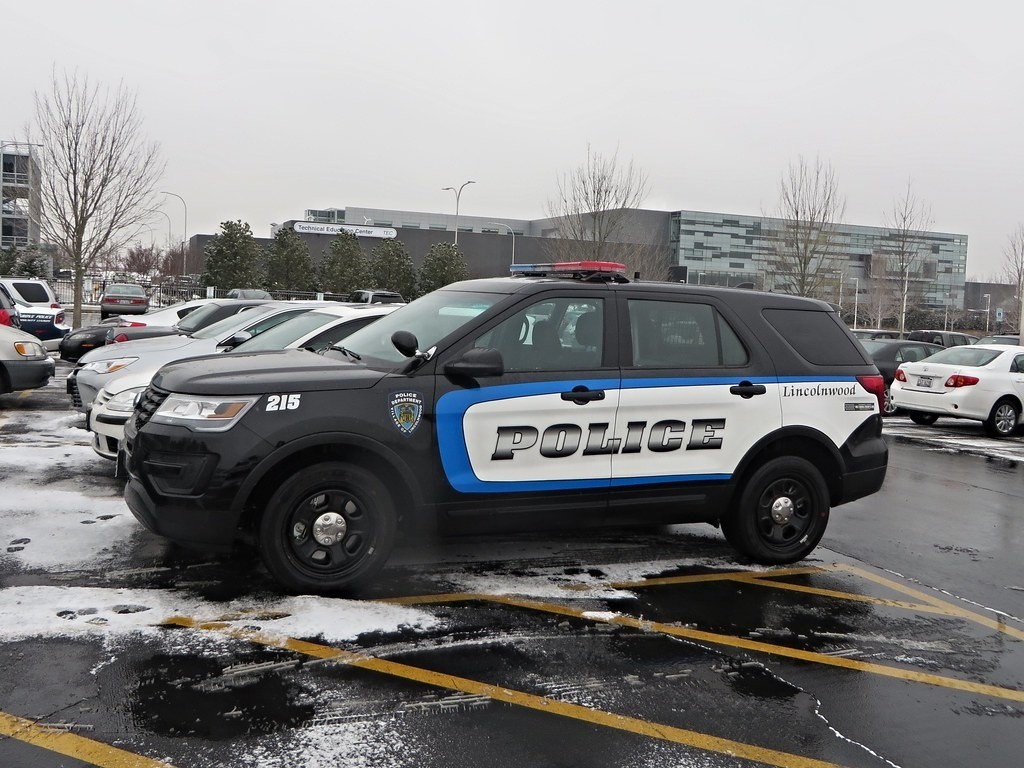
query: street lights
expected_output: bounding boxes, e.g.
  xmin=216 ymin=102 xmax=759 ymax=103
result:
xmin=142 ymin=208 xmax=171 ymax=271
xmin=943 ymin=292 xmax=949 ymax=330
xmin=0 ymin=143 xmax=45 ymax=149
xmin=680 ymin=279 xmax=685 ymax=284
xmin=160 ymin=192 xmax=187 ymax=276
xmin=849 ymin=277 xmax=858 ymax=329
xmin=698 ymin=272 xmax=706 ymax=285
xmin=132 ymin=222 xmax=153 ymax=249
xmin=487 ymin=222 xmax=515 ymax=265
xmin=984 ymin=293 xmax=990 ymax=333
xmin=898 ymin=263 xmax=908 ymax=333
xmin=832 ymin=270 xmax=842 ymax=318
xmin=441 ymin=181 xmax=476 ymax=255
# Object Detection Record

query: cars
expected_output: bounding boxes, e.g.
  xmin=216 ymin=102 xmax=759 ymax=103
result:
xmin=83 ymin=271 xmax=192 ymax=287
xmin=101 ymin=285 xmax=149 ymax=320
xmin=58 ymin=323 xmax=115 ymax=361
xmin=973 ymin=334 xmax=1019 ymax=347
xmin=118 ymin=298 xmax=238 ymax=327
xmin=0 ymin=299 xmax=11 ymax=327
xmin=11 ymin=296 xmax=71 ymax=340
xmin=0 ymin=324 xmax=55 ymax=393
xmin=889 ymin=343 xmax=1024 ymax=438
xmin=849 ymin=329 xmax=910 ymax=341
xmin=66 ymin=302 xmax=374 ymax=414
xmin=226 ymin=288 xmax=273 ymax=301
xmin=859 ymin=339 xmax=947 ymax=417
xmin=85 ymin=301 xmax=536 ymax=461
xmin=104 ymin=301 xmax=269 ymax=348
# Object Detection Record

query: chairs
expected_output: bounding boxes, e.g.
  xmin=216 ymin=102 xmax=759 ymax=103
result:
xmin=523 ymin=321 xmax=562 ymax=371
xmin=904 ymin=350 xmax=917 ymax=362
xmin=566 ymin=310 xmax=602 ymax=368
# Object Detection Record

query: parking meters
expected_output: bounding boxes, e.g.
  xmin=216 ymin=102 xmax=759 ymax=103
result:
xmin=996 ymin=308 xmax=1004 ymax=322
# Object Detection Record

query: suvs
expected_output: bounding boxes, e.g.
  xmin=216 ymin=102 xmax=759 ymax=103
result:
xmin=0 ymin=277 xmax=61 ymax=309
xmin=114 ymin=259 xmax=888 ymax=595
xmin=907 ymin=330 xmax=980 ymax=350
xmin=0 ymin=281 xmax=20 ymax=330
xmin=347 ymin=290 xmax=405 ymax=305
